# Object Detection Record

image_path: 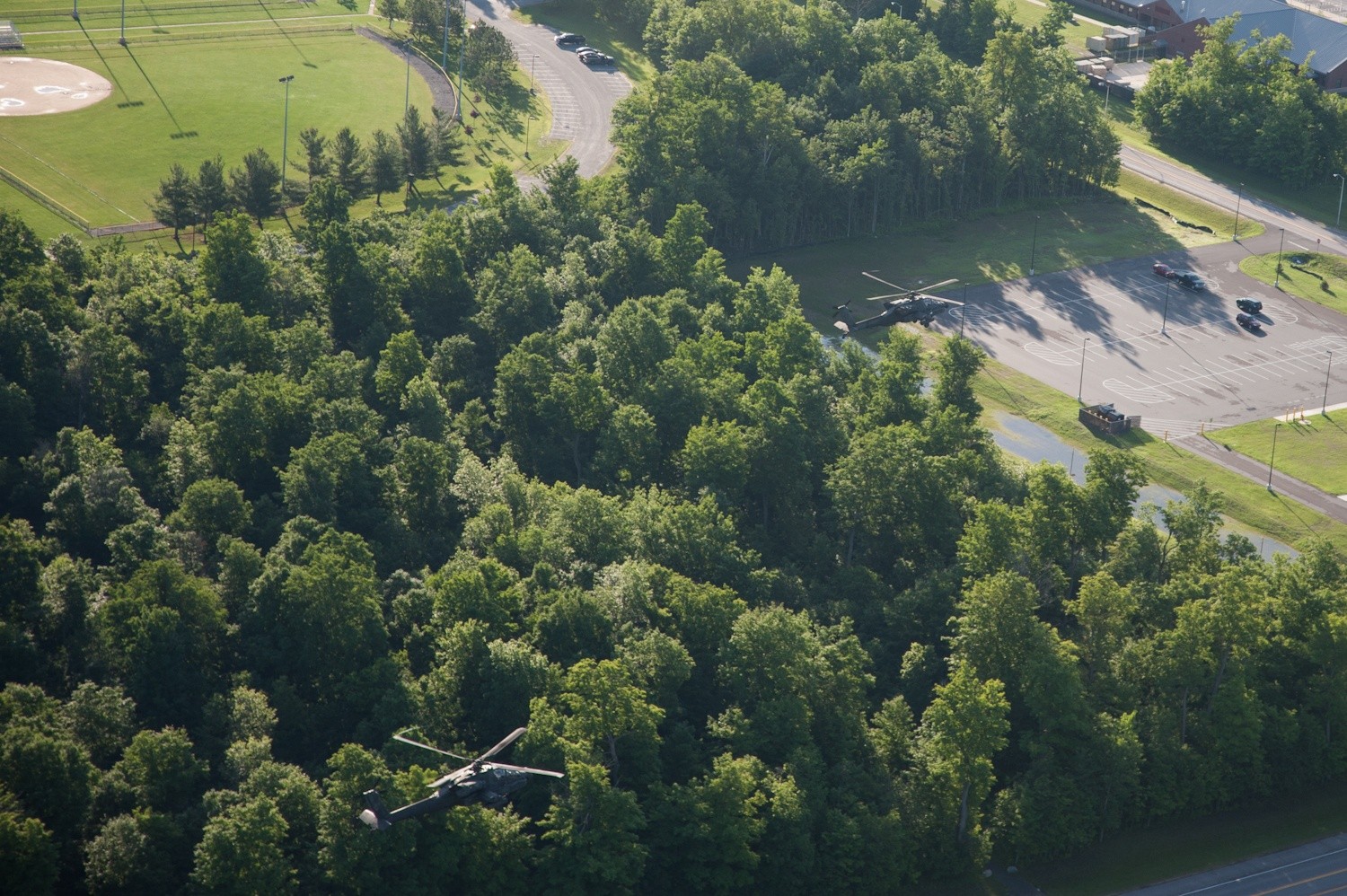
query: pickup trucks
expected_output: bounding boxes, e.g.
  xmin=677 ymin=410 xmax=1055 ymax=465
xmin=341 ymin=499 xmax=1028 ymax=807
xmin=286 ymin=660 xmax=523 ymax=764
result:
xmin=1175 ymin=272 xmax=1206 ymax=290
xmin=554 ymin=33 xmax=586 ymax=45
xmin=583 ymin=55 xmax=614 ymax=66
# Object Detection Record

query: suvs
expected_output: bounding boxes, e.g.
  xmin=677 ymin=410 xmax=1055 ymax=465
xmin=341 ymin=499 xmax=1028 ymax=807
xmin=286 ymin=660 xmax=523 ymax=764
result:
xmin=1237 ymin=298 xmax=1262 ymax=314
xmin=1236 ymin=312 xmax=1261 ymax=330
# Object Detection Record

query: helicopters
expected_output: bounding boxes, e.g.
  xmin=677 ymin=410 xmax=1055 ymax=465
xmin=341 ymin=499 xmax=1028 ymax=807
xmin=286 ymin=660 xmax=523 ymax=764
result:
xmin=833 ymin=272 xmax=966 ymax=339
xmin=358 ymin=727 xmax=565 ymax=832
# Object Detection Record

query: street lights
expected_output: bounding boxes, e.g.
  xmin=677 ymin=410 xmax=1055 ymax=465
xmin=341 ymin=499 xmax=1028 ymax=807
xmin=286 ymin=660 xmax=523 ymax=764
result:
xmin=1162 ymin=278 xmax=1170 ymax=334
xmin=279 ymin=74 xmax=294 ymax=193
xmin=1274 ymin=228 xmax=1284 ymax=288
xmin=1267 ymin=424 xmax=1283 ymax=491
xmin=530 ymin=55 xmax=539 ymax=93
xmin=1029 ymin=216 xmax=1040 ymax=277
xmin=1322 ymin=351 xmax=1332 ymax=416
xmin=1077 ymin=338 xmax=1091 ymax=402
xmin=525 ymin=117 xmax=535 ymax=158
xmin=1098 ymin=82 xmax=1109 ymax=118
xmin=403 ymin=38 xmax=413 ymax=122
xmin=1233 ymin=182 xmax=1245 ymax=241
xmin=961 ymin=283 xmax=971 ymax=341
xmin=1333 ymin=174 xmax=1345 ymax=225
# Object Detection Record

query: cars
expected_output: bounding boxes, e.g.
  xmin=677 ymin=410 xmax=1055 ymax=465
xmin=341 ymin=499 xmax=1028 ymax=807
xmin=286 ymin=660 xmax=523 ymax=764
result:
xmin=575 ymin=46 xmax=605 ymax=58
xmin=1153 ymin=264 xmax=1175 ymax=278
